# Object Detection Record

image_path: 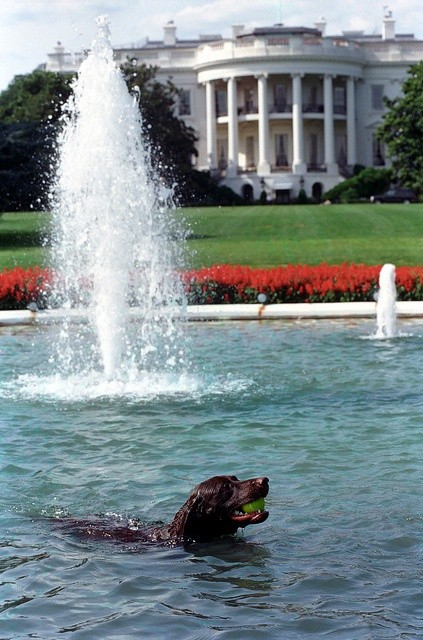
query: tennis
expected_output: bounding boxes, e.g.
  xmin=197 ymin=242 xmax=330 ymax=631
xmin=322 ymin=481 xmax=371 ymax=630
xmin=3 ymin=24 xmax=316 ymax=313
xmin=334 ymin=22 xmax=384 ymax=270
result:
xmin=242 ymin=496 xmax=266 ymax=514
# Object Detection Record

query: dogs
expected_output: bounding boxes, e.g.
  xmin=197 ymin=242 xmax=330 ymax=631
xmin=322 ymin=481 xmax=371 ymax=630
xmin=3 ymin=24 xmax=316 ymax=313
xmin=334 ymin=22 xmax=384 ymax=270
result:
xmin=27 ymin=473 xmax=270 ymax=557
